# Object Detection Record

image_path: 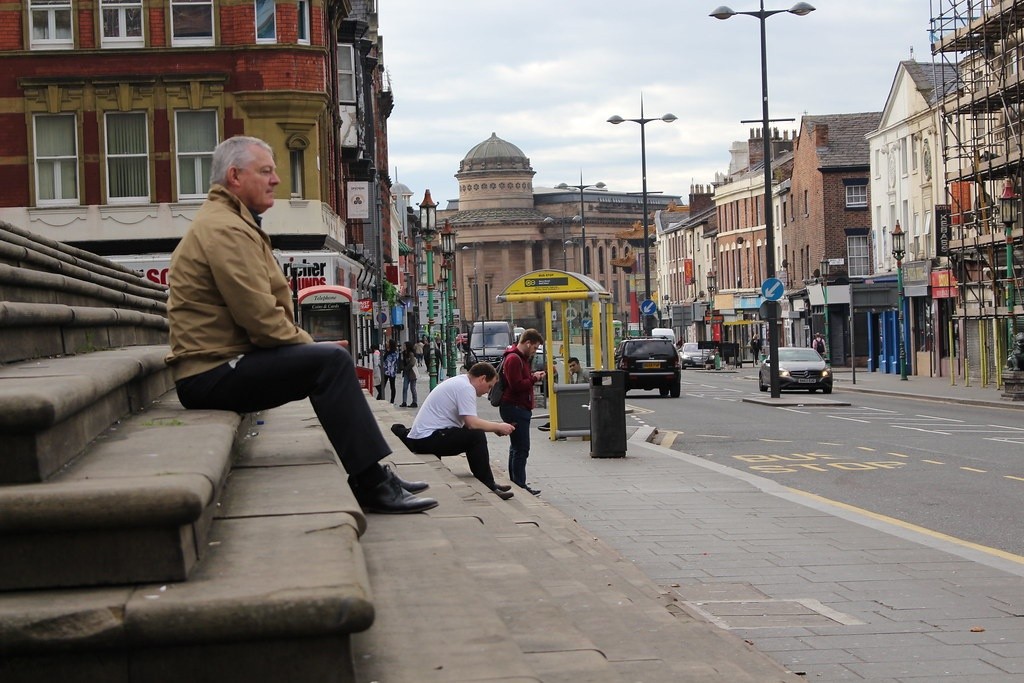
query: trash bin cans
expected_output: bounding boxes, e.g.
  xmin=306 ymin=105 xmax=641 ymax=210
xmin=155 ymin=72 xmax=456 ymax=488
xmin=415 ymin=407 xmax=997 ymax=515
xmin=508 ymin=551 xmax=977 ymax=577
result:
xmin=590 ymin=370 xmax=630 ymax=459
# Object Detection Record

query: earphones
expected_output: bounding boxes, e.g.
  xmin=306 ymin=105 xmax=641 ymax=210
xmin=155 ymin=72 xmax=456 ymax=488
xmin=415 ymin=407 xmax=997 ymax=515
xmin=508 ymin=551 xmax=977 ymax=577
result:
xmin=527 ymin=343 xmax=530 ymax=348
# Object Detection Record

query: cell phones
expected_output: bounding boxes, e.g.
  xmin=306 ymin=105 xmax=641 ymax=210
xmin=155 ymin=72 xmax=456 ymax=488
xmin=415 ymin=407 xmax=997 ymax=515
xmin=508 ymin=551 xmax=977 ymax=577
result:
xmin=540 ymin=373 xmax=544 ymax=375
xmin=496 ymin=423 xmax=516 ymax=437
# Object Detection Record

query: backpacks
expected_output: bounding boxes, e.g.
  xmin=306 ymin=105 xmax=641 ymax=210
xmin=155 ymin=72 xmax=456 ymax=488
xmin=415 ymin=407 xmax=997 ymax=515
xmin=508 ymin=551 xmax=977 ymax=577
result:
xmin=815 ymin=338 xmax=825 ymax=353
xmin=487 ymin=356 xmax=508 ymax=407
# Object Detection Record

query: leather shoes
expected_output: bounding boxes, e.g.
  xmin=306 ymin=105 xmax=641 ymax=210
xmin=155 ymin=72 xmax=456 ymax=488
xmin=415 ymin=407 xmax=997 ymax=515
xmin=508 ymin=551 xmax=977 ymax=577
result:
xmin=383 ymin=462 xmax=430 ymax=494
xmin=354 ymin=474 xmax=439 ymax=514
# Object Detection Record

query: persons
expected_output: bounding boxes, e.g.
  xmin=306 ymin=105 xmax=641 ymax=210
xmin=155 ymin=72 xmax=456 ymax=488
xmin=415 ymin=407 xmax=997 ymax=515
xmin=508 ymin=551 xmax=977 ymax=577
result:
xmin=812 ymin=333 xmax=827 ymax=360
xmin=750 ymin=334 xmax=762 ymax=365
xmin=167 ymin=134 xmax=438 ymax=514
xmin=399 ymin=341 xmax=419 ymax=407
xmin=413 ymin=333 xmax=443 ymax=384
xmin=498 ymin=328 xmax=546 ymax=494
xmin=375 ymin=339 xmax=398 ymax=403
xmin=537 ymin=357 xmax=590 ymax=440
xmin=406 ymin=362 xmax=514 ymax=499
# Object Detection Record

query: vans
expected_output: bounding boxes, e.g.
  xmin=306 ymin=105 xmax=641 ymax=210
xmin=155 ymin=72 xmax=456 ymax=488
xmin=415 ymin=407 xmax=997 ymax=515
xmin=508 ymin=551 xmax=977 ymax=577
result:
xmin=652 ymin=327 xmax=677 ymax=354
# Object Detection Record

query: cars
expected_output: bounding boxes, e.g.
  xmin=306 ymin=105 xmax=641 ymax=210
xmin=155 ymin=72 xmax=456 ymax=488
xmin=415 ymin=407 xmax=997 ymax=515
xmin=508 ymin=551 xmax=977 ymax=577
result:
xmin=759 ymin=347 xmax=834 ymax=394
xmin=455 ymin=320 xmax=559 ymax=396
xmin=679 ymin=341 xmax=715 ymax=370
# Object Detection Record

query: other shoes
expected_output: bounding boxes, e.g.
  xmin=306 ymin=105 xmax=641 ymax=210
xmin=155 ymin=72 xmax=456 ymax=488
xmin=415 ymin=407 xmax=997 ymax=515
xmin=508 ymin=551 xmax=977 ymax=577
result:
xmin=377 ymin=394 xmax=382 ymax=400
xmin=400 ymin=402 xmax=407 ymax=407
xmin=408 ymin=402 xmax=417 ymax=407
xmin=494 ymin=488 xmax=514 ymax=500
xmin=497 ymin=485 xmax=511 ymax=491
xmin=528 ymin=489 xmax=542 ymax=496
xmin=390 ymin=398 xmax=394 ymax=403
xmin=538 ymin=422 xmax=550 ymax=431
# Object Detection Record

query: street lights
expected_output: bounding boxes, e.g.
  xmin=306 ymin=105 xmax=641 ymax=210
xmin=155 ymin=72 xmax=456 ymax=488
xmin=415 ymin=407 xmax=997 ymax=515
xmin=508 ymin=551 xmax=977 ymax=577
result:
xmin=890 ymin=219 xmax=909 ymax=381
xmin=462 ymin=241 xmax=478 ymax=322
xmin=996 ymin=176 xmax=1020 ymax=358
xmin=419 ymin=189 xmax=439 ymax=393
xmin=706 ymin=269 xmax=716 ymax=340
xmin=564 ymin=238 xmax=581 ymax=274
xmin=543 ymin=210 xmax=583 ymax=271
xmin=551 ymin=169 xmax=608 ymax=366
xmin=707 ymin=1 xmax=817 ymax=397
xmin=605 ymin=91 xmax=678 ymax=299
xmin=817 ymin=254 xmax=832 ymax=367
xmin=438 ymin=215 xmax=458 ymax=377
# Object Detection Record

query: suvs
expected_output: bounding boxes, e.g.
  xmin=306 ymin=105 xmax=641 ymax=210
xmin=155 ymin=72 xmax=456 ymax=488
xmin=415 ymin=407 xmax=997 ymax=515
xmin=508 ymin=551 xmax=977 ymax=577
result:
xmin=615 ymin=336 xmax=681 ymax=399
